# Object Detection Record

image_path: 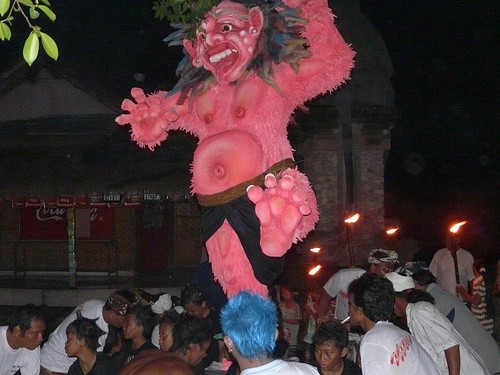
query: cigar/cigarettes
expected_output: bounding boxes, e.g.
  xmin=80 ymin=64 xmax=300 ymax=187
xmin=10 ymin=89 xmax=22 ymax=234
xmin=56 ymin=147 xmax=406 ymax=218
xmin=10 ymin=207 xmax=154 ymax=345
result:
xmin=342 ymin=316 xmax=350 ymax=325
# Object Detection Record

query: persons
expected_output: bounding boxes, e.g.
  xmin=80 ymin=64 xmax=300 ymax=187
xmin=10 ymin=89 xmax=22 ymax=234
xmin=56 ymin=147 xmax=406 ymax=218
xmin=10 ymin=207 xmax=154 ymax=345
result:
xmin=0 ymin=223 xmax=500 ymax=375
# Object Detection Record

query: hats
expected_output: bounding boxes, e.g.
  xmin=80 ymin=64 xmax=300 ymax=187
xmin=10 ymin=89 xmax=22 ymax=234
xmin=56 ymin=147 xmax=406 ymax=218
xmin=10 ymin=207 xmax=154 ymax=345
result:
xmin=395 ymin=261 xmax=429 ymax=275
xmin=384 ymin=271 xmax=415 ymax=292
